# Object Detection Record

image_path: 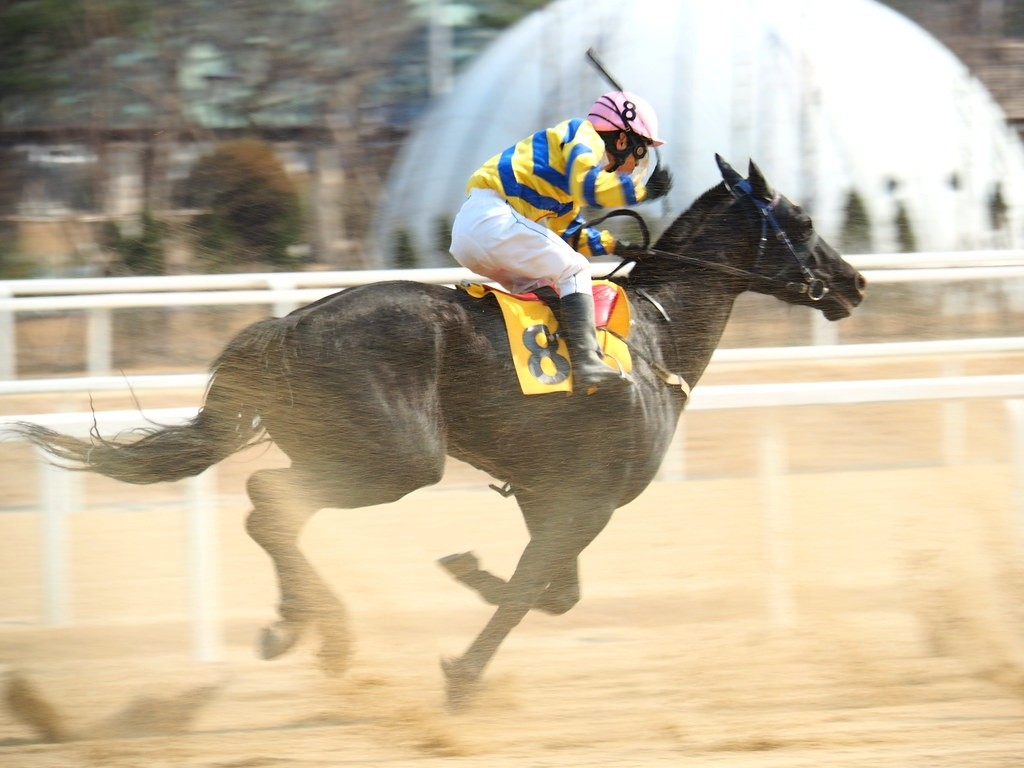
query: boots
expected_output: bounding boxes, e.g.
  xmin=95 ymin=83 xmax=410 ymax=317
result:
xmin=558 ymin=292 xmax=635 ymax=396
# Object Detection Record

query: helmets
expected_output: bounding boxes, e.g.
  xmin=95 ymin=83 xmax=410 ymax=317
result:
xmin=586 ymin=92 xmax=663 ymax=148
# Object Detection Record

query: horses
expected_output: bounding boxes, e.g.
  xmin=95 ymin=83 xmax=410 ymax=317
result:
xmin=1 ymin=152 xmax=866 ymax=713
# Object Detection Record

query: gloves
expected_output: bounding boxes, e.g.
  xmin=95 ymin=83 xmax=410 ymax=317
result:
xmin=645 ymin=163 xmax=673 ymax=200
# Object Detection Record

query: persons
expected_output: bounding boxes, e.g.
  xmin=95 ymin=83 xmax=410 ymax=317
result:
xmin=447 ymin=91 xmax=675 ymax=388
